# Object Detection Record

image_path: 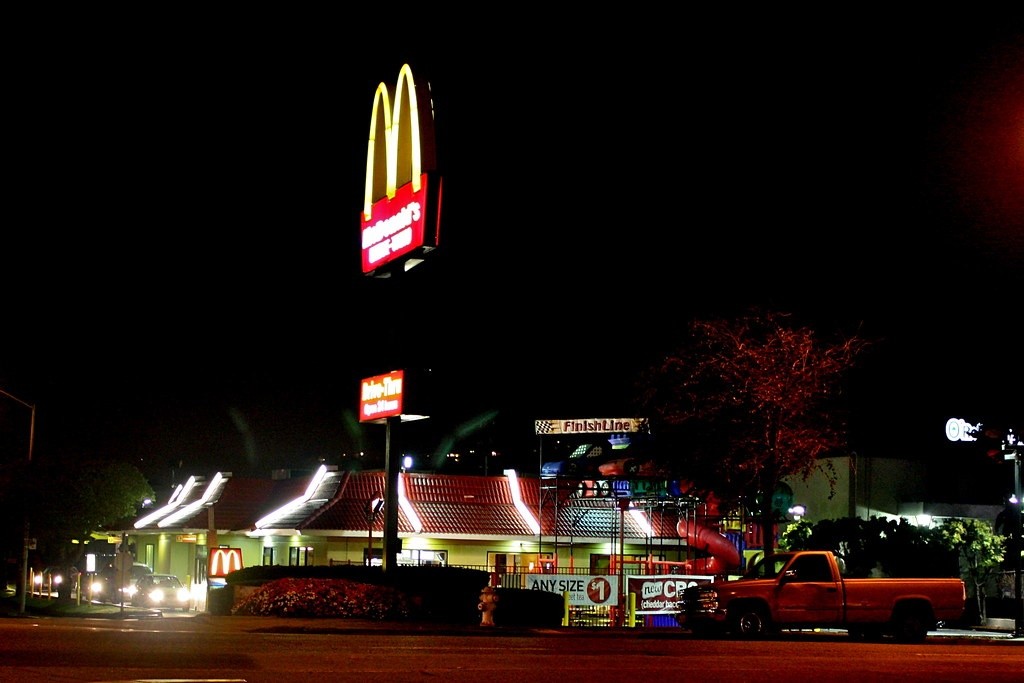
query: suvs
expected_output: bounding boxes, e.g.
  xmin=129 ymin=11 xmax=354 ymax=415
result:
xmin=100 ymin=560 xmax=154 ymax=603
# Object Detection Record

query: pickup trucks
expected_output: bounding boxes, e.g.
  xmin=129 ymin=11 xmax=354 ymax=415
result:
xmin=676 ymin=552 xmax=970 ymax=640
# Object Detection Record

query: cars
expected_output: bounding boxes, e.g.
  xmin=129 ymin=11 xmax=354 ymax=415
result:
xmin=34 ymin=566 xmax=74 ymax=594
xmin=75 ymin=571 xmax=105 ymax=599
xmin=131 ymin=575 xmax=192 ymax=611
